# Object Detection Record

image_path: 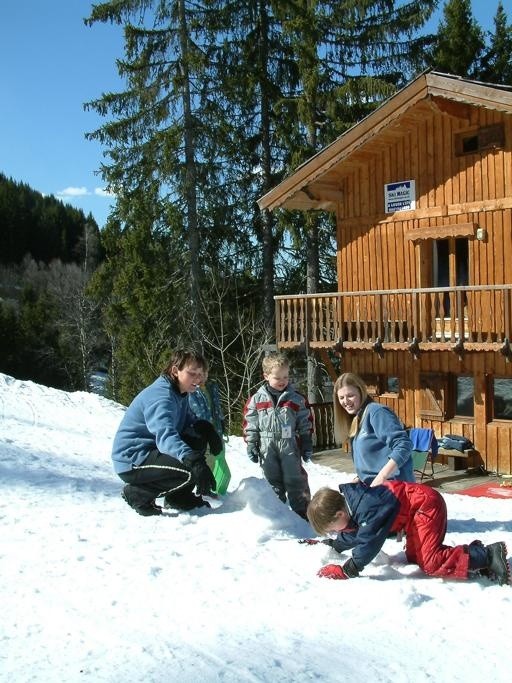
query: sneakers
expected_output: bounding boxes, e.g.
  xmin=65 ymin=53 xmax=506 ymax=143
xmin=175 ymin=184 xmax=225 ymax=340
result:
xmin=486 ymin=540 xmax=510 ymax=587
xmin=468 ymin=539 xmax=490 ymax=575
xmin=164 ymin=491 xmax=213 ymax=513
xmin=120 ymin=483 xmax=167 ymax=518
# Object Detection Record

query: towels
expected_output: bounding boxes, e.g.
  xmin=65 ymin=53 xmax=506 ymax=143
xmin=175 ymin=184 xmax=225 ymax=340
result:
xmin=408 ymin=427 xmax=440 ymax=458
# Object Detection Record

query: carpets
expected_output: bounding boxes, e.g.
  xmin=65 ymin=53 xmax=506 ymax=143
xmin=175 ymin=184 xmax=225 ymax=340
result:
xmin=445 ymin=479 xmax=512 ymax=500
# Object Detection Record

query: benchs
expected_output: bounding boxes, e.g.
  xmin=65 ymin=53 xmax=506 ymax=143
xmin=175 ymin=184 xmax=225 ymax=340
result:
xmin=427 ymin=446 xmax=477 ymax=472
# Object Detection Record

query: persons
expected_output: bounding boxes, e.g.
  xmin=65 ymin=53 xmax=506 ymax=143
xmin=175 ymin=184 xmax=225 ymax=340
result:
xmin=110 ymin=346 xmax=222 ymax=516
xmin=243 ymin=352 xmax=314 ymax=521
xmin=299 ymin=479 xmax=511 ymax=586
xmin=332 ymin=371 xmax=416 ymax=540
xmin=189 ymin=353 xmax=225 ymax=499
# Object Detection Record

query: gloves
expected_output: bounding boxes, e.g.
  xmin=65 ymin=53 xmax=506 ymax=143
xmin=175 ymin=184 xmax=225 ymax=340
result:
xmin=317 ymin=562 xmax=348 ymax=579
xmin=192 ymin=418 xmax=225 ymax=457
xmin=184 ymin=450 xmax=218 ymax=496
xmin=246 ymin=442 xmax=260 ymax=465
xmin=299 ymin=436 xmax=315 ymax=462
